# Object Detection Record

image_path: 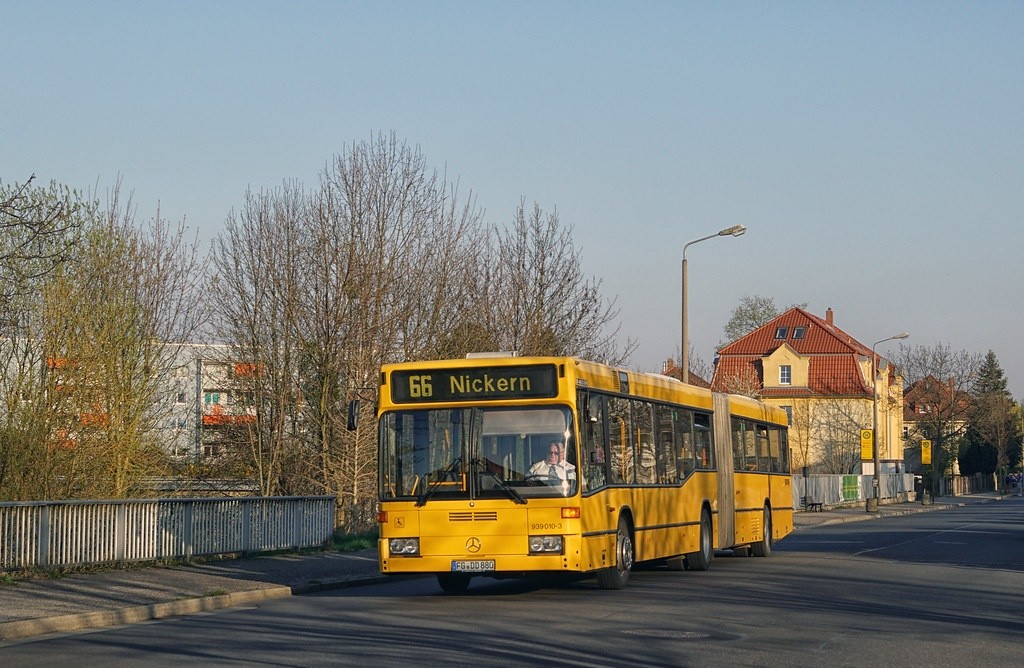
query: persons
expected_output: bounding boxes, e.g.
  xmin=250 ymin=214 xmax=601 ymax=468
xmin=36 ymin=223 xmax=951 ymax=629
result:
xmin=525 ymin=442 xmax=588 ymax=496
xmin=1005 ymin=471 xmax=1022 ymax=497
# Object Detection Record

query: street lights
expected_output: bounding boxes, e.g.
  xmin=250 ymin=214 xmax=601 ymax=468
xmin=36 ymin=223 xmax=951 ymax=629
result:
xmin=872 ymin=330 xmax=909 ymax=508
xmin=679 ymin=224 xmax=747 ymax=385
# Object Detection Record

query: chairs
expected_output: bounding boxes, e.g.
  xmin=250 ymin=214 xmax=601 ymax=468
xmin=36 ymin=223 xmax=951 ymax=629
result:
xmin=670 ymin=456 xmax=782 ymax=473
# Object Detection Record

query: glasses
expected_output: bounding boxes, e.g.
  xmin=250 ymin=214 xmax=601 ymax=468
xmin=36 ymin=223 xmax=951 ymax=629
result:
xmin=548 ymin=451 xmax=559 ymax=455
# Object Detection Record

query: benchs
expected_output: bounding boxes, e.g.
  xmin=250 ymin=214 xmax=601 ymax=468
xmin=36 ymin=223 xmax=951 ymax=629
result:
xmin=800 ymin=496 xmax=823 ymax=511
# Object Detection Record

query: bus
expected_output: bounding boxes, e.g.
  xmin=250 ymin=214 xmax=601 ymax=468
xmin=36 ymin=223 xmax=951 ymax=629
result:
xmin=347 ymin=349 xmax=795 ymax=595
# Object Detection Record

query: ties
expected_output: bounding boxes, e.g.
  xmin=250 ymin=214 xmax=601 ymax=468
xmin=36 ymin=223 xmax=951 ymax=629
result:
xmin=548 ymin=466 xmax=559 ymax=480
